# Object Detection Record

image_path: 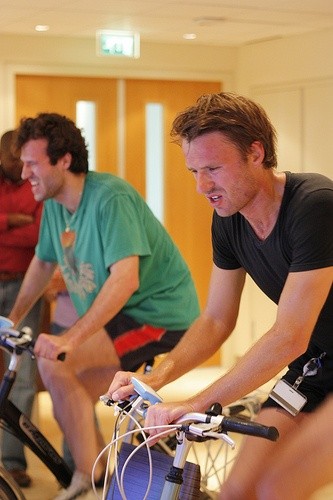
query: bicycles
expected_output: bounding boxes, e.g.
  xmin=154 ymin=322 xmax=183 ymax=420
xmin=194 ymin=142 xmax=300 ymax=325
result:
xmin=0 ymin=315 xmax=266 ymax=500
xmin=91 ymin=377 xmax=281 ymax=499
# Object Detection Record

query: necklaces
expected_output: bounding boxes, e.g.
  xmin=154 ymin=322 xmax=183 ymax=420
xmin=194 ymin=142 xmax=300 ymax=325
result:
xmin=60 ymin=203 xmax=79 ymax=249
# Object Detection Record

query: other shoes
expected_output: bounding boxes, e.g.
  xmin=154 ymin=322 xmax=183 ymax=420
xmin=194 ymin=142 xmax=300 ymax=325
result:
xmin=10 ymin=468 xmax=32 ymax=488
xmin=52 ymin=460 xmax=108 ymax=500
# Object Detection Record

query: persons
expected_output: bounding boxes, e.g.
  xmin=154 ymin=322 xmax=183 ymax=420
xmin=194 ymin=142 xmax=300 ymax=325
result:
xmin=0 ymin=114 xmax=200 ymax=500
xmin=107 ymin=93 xmax=333 ymax=500
